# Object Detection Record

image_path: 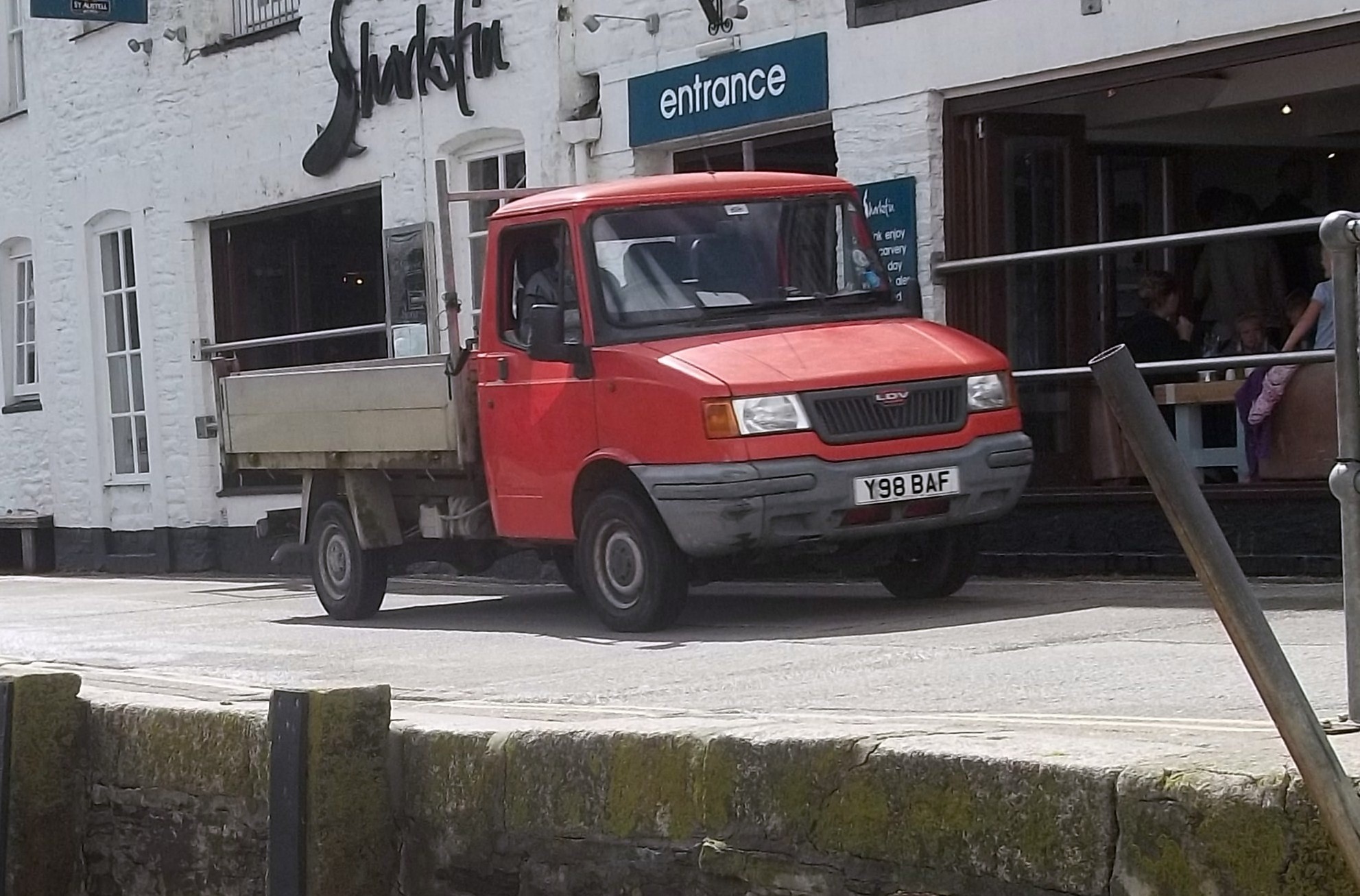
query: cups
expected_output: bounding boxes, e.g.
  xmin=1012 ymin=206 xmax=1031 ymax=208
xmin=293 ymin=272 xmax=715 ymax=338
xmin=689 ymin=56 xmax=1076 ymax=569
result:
xmin=1226 ymin=369 xmax=1235 ymax=381
xmin=1244 ymin=367 xmax=1255 ymax=377
xmin=1198 ymin=370 xmax=1216 ymax=382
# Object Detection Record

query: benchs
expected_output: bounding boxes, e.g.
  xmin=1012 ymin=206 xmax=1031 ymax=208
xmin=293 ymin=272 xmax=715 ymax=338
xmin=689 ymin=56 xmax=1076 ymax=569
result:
xmin=0 ymin=514 xmax=54 ymax=574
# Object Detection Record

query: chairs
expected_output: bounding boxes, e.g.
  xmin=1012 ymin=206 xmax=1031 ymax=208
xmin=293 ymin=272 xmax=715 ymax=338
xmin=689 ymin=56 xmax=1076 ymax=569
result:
xmin=689 ymin=237 xmax=752 ymax=290
xmin=516 ymin=245 xmax=559 ymax=310
xmin=1234 ymin=361 xmax=1338 ymax=479
xmin=623 ymin=241 xmax=681 ymax=284
xmin=1087 ymin=387 xmax=1145 ymax=488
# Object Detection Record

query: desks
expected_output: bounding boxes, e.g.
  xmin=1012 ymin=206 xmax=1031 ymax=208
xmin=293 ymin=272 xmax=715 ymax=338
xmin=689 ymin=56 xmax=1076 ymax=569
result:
xmin=1155 ymin=380 xmax=1250 ymax=483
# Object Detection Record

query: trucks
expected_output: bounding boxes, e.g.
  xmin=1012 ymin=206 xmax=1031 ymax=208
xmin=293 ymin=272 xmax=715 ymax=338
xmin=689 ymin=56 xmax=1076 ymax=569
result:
xmin=220 ymin=160 xmax=1033 ymax=635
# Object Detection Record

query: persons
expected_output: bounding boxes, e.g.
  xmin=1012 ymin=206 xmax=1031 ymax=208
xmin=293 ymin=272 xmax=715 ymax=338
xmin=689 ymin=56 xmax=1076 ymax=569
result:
xmin=520 ymin=222 xmax=624 ymax=342
xmin=1119 ymin=205 xmax=1334 ymax=483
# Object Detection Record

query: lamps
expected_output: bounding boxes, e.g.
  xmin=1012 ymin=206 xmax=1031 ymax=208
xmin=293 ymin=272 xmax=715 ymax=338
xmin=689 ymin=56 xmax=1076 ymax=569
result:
xmin=128 ymin=39 xmax=153 ymax=54
xmin=584 ymin=14 xmax=659 ymax=33
xmin=729 ymin=0 xmax=748 ymax=19
xmin=163 ymin=25 xmax=186 ymax=43
